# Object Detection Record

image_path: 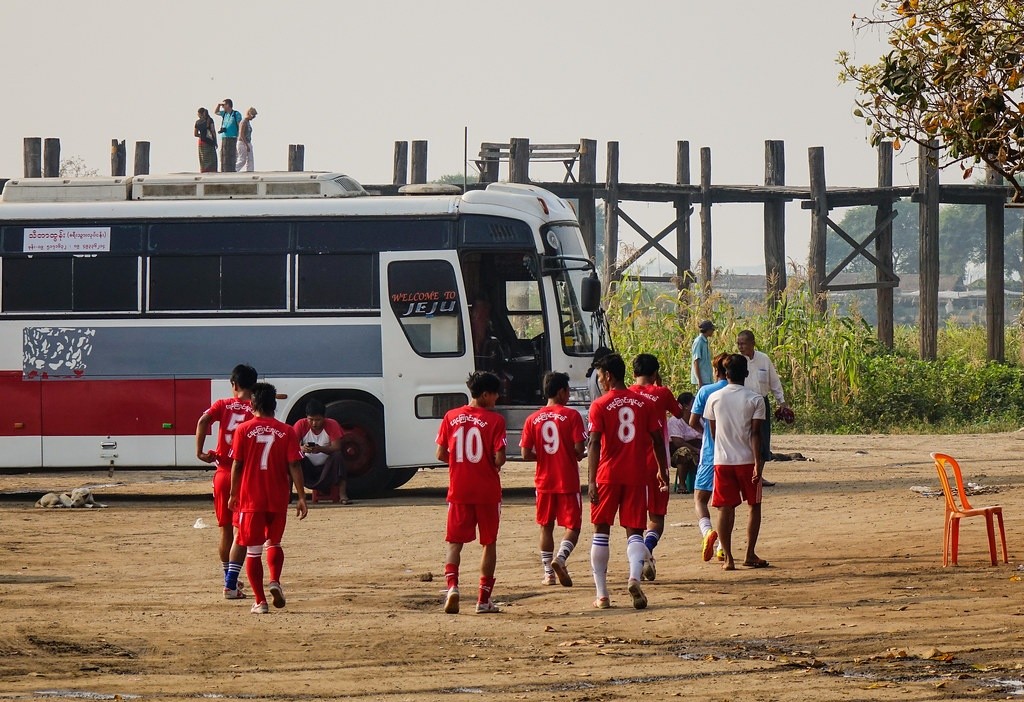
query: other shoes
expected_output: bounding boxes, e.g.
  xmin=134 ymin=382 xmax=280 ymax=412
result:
xmin=677 ymin=483 xmax=688 ymax=494
xmin=762 ymin=479 xmax=775 ymax=486
xmin=338 ymin=497 xmax=352 ymax=504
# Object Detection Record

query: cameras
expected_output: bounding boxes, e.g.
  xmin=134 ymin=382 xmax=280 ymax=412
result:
xmin=218 ymin=127 xmax=227 ymax=134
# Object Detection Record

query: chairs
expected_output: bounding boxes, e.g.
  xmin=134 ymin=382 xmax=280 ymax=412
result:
xmin=930 ymin=452 xmax=1008 ymax=569
xmin=489 ymin=333 xmax=535 ymax=377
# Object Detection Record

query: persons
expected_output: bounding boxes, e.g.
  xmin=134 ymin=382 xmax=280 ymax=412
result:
xmin=235 ymin=107 xmax=257 ymax=171
xmin=194 ymin=107 xmax=218 ymax=173
xmin=628 ymin=353 xmax=683 ymax=580
xmin=436 ymin=371 xmax=508 ymax=612
xmin=196 ymin=363 xmax=309 ymax=613
xmin=518 ymin=372 xmax=585 ymax=586
xmin=293 ymin=401 xmax=352 ymax=506
xmin=215 ymin=98 xmax=243 ymax=171
xmin=587 ymin=318 xmax=786 ymax=612
xmin=702 ymin=354 xmax=768 ymax=569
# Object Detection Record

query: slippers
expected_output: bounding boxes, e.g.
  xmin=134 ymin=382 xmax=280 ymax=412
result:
xmin=743 ymin=554 xmax=769 ymax=567
xmin=721 ymin=561 xmax=735 ymax=570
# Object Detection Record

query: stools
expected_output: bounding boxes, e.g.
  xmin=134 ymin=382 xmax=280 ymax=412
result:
xmin=674 ymin=467 xmax=696 ymax=493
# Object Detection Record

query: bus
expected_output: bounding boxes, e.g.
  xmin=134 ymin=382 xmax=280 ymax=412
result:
xmin=1 ymin=172 xmax=615 ymax=500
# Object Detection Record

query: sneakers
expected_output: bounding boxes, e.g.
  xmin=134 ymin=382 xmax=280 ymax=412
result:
xmin=703 ymin=529 xmax=717 ymax=562
xmin=223 ymin=587 xmax=246 ymax=598
xmin=223 ymin=578 xmax=244 ymax=590
xmin=593 ymin=597 xmax=610 ymax=608
xmin=640 ymin=552 xmax=657 ymax=581
xmin=628 ymin=577 xmax=647 ymax=609
xmin=542 ymin=573 xmax=555 ymax=585
xmin=269 ymin=580 xmax=286 ymax=608
xmin=717 ymin=549 xmax=725 ymax=561
xmin=443 ymin=586 xmax=459 ymax=613
xmin=251 ymin=601 xmax=268 ymax=613
xmin=476 ymin=597 xmax=499 ymax=612
xmin=551 ymin=557 xmax=573 ymax=586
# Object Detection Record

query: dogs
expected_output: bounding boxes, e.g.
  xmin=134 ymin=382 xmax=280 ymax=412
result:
xmin=34 ymin=488 xmax=109 ymax=508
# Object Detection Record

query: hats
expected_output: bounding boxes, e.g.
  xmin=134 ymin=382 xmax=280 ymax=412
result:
xmin=699 ymin=320 xmax=715 ymax=331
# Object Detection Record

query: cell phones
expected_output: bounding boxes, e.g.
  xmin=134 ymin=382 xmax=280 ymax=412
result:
xmin=308 ymin=442 xmax=315 ymax=448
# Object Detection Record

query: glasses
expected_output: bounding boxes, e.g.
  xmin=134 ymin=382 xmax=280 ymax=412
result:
xmin=713 ymin=329 xmax=715 ymax=331
xmin=249 ymin=112 xmax=255 ymax=118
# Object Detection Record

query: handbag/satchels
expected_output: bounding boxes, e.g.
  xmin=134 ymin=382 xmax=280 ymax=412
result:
xmin=206 ymin=118 xmax=215 ymax=144
